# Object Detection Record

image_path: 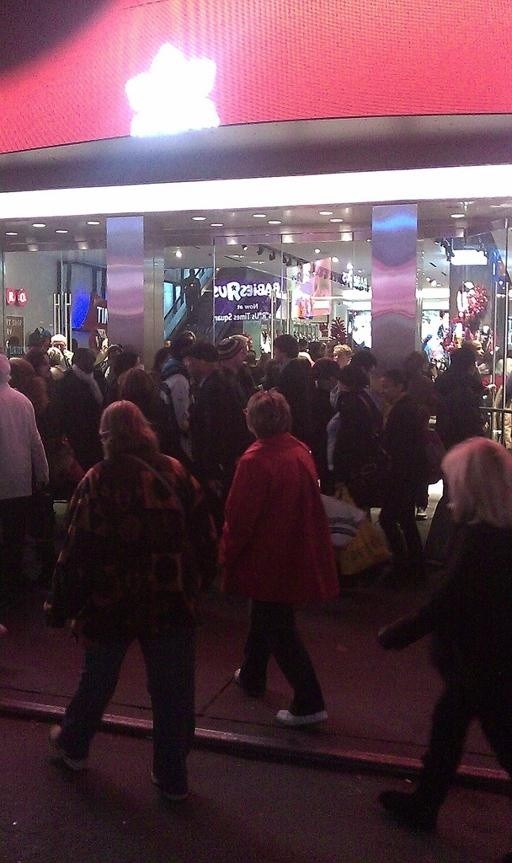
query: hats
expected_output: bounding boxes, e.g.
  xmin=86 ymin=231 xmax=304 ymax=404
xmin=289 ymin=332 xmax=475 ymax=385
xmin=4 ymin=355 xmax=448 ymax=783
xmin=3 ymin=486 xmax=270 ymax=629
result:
xmin=182 ymin=342 xmax=217 ymax=363
xmin=217 ymin=336 xmax=243 ymax=359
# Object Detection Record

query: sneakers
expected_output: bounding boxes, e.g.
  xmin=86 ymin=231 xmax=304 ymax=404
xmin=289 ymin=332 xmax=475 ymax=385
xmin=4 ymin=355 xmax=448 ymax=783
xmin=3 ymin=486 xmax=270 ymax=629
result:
xmin=380 ymin=790 xmax=437 ymax=831
xmin=416 ymin=507 xmax=428 ymax=521
xmin=150 ymin=771 xmax=189 ymax=801
xmin=49 ymin=726 xmax=88 ymax=770
xmin=233 ymin=669 xmax=263 ymax=697
xmin=277 ymin=709 xmax=328 ymax=727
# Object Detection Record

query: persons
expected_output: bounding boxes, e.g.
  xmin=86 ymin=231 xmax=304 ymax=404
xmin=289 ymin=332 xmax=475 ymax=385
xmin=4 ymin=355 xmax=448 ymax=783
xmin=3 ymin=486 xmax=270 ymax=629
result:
xmin=44 ymin=400 xmax=218 ymax=802
xmin=376 ymin=435 xmax=511 ymax=830
xmin=0 ymin=328 xmax=512 ymax=594
xmin=219 ymin=388 xmax=341 ymax=727
xmin=182 ymin=269 xmax=202 ymax=327
